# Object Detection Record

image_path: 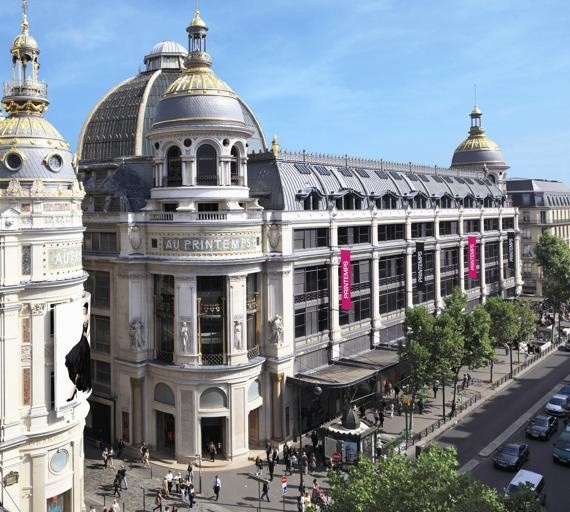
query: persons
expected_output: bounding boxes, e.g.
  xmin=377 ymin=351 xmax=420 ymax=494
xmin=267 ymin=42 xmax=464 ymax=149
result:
xmin=269 ymin=314 xmax=282 ymax=344
xmin=65 ymin=302 xmax=91 ymax=402
xmin=234 ymin=321 xmax=242 ymax=351
xmin=181 ymin=322 xmax=189 ymax=353
xmin=132 ymin=320 xmax=143 ymax=349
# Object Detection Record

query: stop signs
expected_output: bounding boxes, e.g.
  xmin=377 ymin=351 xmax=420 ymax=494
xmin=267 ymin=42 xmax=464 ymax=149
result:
xmin=333 ymin=452 xmax=341 ymax=461
xmin=280 ymin=476 xmax=287 ymax=483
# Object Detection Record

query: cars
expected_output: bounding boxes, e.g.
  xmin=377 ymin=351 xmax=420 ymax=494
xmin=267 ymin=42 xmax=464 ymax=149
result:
xmin=525 ymin=384 xmax=570 ymax=467
xmin=502 ymin=469 xmax=546 ymax=507
xmin=492 ymin=441 xmax=530 ymax=473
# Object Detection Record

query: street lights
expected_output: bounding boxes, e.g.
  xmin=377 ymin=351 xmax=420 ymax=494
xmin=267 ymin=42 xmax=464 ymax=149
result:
xmin=294 ymin=373 xmax=340 ymax=495
xmin=140 ymin=486 xmax=145 ymax=512
xmin=194 ymin=454 xmax=201 ymax=494
